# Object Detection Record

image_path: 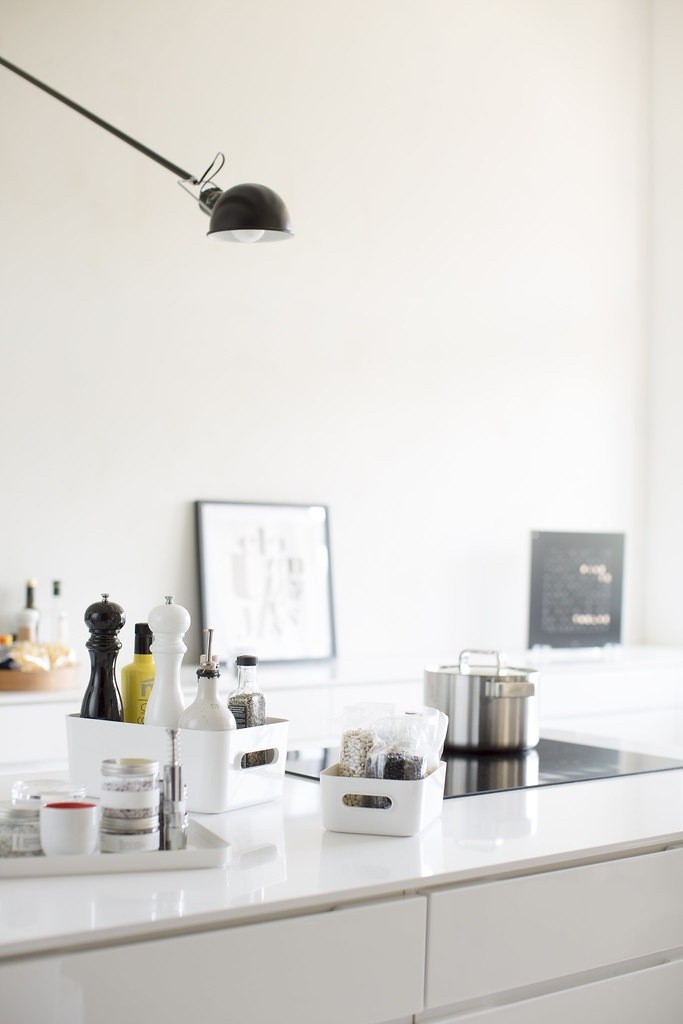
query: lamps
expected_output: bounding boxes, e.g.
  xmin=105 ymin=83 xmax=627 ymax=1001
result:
xmin=1 ymin=56 xmax=297 ymax=238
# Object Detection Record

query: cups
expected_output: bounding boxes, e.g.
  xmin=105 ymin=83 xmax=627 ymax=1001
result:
xmin=0 ymin=800 xmax=44 ymax=857
xmin=101 ymin=760 xmax=159 ymax=853
xmin=10 ymin=780 xmax=87 ymax=801
xmin=41 ymin=801 xmax=97 ymax=856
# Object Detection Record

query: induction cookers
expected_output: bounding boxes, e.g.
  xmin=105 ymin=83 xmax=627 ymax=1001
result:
xmin=279 ymin=738 xmax=683 ymax=800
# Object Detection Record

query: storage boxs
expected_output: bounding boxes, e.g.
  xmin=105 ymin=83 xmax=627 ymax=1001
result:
xmin=320 ymin=758 xmax=447 ymax=836
xmin=67 ymin=712 xmax=291 ymax=814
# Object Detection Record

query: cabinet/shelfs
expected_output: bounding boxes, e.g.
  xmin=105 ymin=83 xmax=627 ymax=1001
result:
xmin=0 ymin=740 xmax=683 ymax=1024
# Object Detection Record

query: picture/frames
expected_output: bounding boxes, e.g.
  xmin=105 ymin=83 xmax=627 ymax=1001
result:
xmin=194 ymin=497 xmax=335 ymax=666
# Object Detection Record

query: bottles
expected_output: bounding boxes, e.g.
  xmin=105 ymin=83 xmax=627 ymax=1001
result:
xmin=143 ymin=595 xmax=191 ymax=726
xmin=13 ymin=580 xmax=39 ymax=641
xmin=228 ymin=655 xmax=265 ymax=768
xmin=45 ymin=580 xmax=67 ymax=643
xmin=179 ymin=670 xmax=235 ymax=731
xmin=79 ymin=594 xmax=124 ymax=722
xmin=119 ymin=623 xmax=157 ymax=724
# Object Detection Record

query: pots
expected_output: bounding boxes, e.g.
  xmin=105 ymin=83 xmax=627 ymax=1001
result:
xmin=427 ymin=647 xmax=540 ymax=753
